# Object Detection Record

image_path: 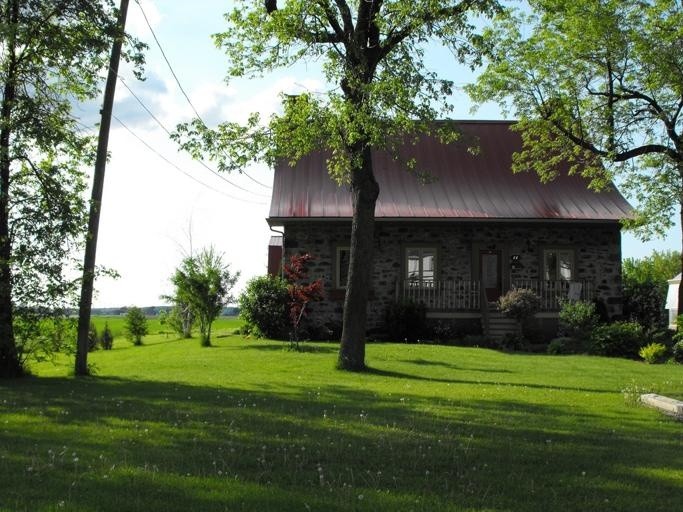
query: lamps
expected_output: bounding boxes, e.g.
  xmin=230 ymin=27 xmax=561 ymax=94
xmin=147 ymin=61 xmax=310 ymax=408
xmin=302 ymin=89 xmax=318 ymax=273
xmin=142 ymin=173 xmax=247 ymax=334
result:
xmin=511 ymin=255 xmax=521 ymax=270
